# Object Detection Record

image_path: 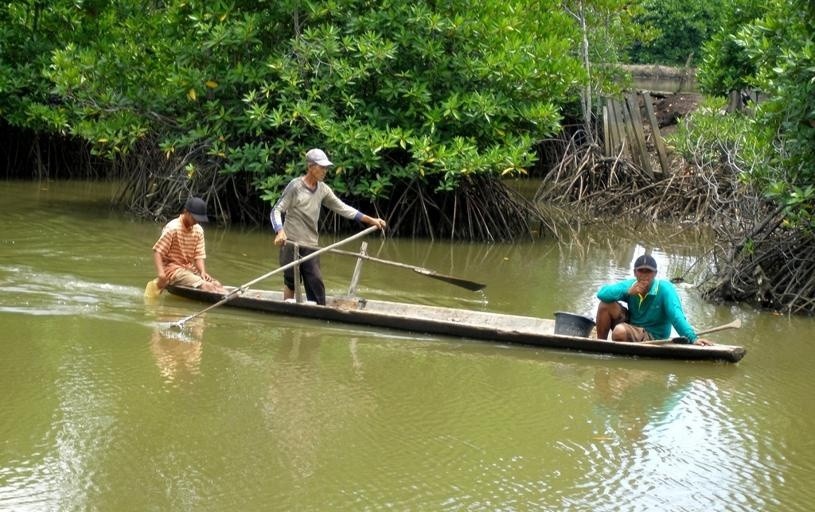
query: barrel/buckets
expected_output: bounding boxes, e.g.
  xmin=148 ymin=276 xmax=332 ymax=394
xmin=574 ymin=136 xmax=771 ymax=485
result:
xmin=553 ymin=311 xmax=595 ymax=336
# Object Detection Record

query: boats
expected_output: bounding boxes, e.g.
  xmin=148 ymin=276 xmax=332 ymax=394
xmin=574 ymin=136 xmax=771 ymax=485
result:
xmin=164 ymin=277 xmax=750 ymax=366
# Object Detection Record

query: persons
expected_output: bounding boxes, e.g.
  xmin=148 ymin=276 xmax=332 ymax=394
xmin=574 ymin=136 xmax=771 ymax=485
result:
xmin=596 ymin=256 xmax=714 ymax=346
xmin=151 ymin=308 xmax=204 ymax=375
xmin=152 ymin=197 xmax=228 ymax=293
xmin=266 ymin=330 xmax=375 ymax=477
xmin=593 ymin=368 xmax=691 ymax=441
xmin=270 ymin=149 xmax=387 ymax=305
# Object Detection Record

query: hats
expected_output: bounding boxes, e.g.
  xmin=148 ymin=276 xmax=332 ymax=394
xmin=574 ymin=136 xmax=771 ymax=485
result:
xmin=186 ymin=198 xmax=208 ymax=222
xmin=635 ymin=255 xmax=657 ymax=272
xmin=306 ymin=148 xmax=334 ymax=167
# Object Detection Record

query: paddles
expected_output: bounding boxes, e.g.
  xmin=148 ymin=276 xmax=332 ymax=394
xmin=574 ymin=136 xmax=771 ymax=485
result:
xmin=285 ymin=240 xmax=486 ymax=291
xmin=170 ymin=224 xmax=379 ymax=328
